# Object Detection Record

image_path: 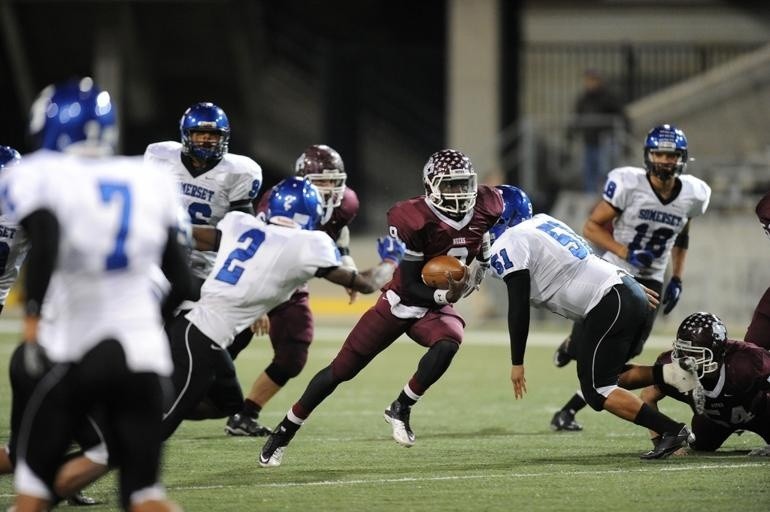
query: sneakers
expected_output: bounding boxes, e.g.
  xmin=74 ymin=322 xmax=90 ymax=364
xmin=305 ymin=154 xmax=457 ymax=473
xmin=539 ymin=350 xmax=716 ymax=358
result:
xmin=224 ymin=415 xmax=269 ymax=436
xmin=550 ymin=411 xmax=581 ymax=432
xmin=554 ymin=337 xmax=573 ymax=366
xmin=653 ymin=356 xmax=706 ymax=416
xmin=259 ymin=427 xmax=289 ymax=467
xmin=385 ymin=402 xmax=415 ymax=447
xmin=69 ymin=490 xmax=94 ymax=505
xmin=639 ymin=424 xmax=694 ymax=460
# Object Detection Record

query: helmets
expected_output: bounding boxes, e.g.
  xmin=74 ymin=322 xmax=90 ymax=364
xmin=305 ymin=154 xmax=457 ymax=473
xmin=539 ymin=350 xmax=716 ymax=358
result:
xmin=423 ymin=151 xmax=478 ymax=214
xmin=0 ymin=146 xmax=20 ymax=173
xmin=644 ymin=124 xmax=687 ymax=176
xmin=296 ymin=145 xmax=346 ymax=207
xmin=483 ymin=184 xmax=532 ymax=243
xmin=31 ymin=80 xmax=115 ymax=149
xmin=267 ymin=177 xmax=323 ymax=231
xmin=180 ymin=103 xmax=229 ymax=158
xmin=674 ymin=314 xmax=727 ymax=378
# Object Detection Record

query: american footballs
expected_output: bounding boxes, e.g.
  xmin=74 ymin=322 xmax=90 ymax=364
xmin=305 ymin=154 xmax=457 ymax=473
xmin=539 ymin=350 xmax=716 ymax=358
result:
xmin=420 ymin=256 xmax=466 ymax=290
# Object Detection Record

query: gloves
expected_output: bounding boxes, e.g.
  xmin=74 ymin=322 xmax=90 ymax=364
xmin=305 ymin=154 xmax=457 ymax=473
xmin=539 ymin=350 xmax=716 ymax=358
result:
xmin=378 ymin=235 xmax=406 ymax=265
xmin=663 ymin=276 xmax=683 ymax=314
xmin=626 ymin=249 xmax=654 ymax=268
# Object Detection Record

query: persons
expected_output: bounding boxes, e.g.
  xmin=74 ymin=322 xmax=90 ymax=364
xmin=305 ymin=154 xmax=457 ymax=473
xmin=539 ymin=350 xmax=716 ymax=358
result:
xmin=7 ymin=173 xmax=406 ymax=512
xmin=640 ymin=310 xmax=769 ymax=452
xmin=490 ymin=185 xmax=697 ymax=462
xmin=1 ymin=77 xmax=195 ymax=512
xmin=226 ymin=144 xmax=359 ymax=437
xmin=257 ymin=149 xmax=504 ymax=469
xmin=143 ymin=103 xmax=263 ymax=301
xmin=0 ymin=144 xmax=28 ymax=314
xmin=551 ymin=123 xmax=713 ymax=433
